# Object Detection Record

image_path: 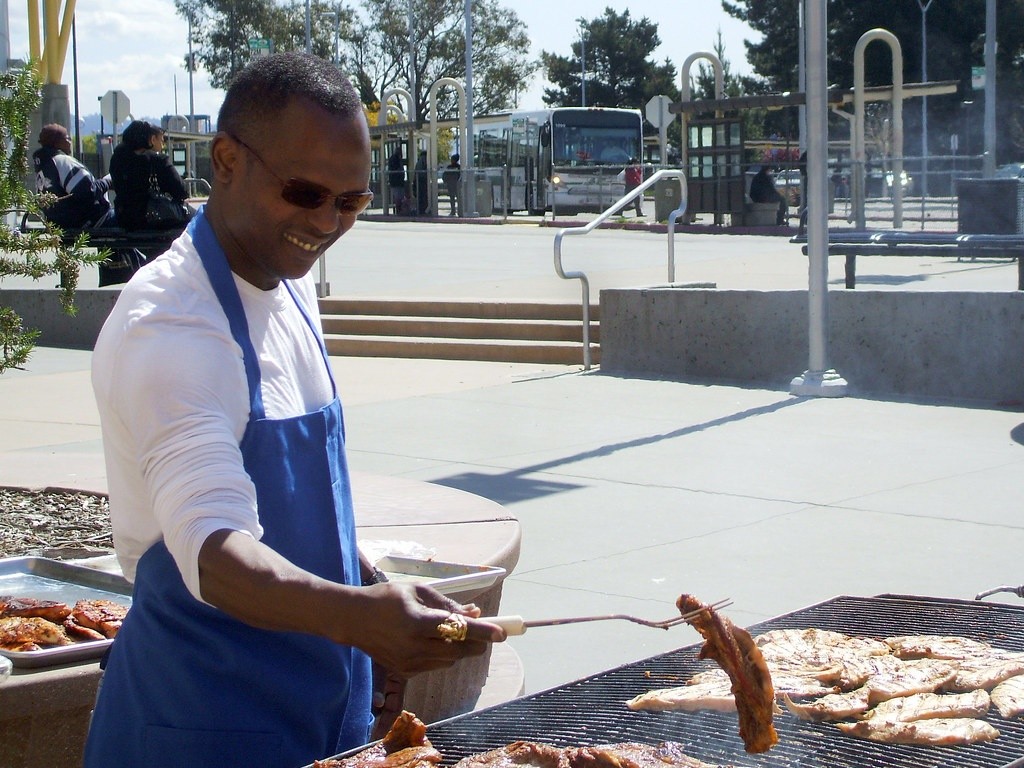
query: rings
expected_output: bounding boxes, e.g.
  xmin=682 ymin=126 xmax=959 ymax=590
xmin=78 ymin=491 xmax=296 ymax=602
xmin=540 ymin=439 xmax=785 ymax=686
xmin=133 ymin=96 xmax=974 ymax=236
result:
xmin=436 ymin=614 xmax=469 ymax=643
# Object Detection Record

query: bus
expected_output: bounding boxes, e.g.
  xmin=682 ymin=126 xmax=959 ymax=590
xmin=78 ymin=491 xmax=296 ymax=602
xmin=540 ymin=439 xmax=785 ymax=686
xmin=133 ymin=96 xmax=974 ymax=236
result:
xmin=472 ymin=105 xmax=646 ymax=215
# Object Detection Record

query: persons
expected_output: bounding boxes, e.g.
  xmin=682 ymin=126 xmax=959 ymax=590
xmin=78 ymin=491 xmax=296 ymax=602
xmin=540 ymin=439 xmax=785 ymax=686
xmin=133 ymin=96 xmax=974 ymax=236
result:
xmin=601 ymin=136 xmax=633 ymax=165
xmin=443 ymin=152 xmax=464 ymax=218
xmin=750 ymin=161 xmax=790 ymax=226
xmin=413 ymin=150 xmax=430 ymax=214
xmin=797 ymin=148 xmax=808 ymax=213
xmin=33 ymin=123 xmax=114 ymax=287
xmin=143 ymin=124 xmax=175 ymax=264
xmin=109 ymin=120 xmax=201 ymax=264
xmin=389 ymin=146 xmax=408 ymax=217
xmin=620 ymin=157 xmax=648 ymax=219
xmin=80 ymin=50 xmax=511 ymax=768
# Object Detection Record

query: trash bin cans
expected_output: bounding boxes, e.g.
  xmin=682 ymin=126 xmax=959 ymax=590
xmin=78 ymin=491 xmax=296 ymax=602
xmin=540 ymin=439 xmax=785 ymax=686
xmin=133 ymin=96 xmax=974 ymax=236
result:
xmin=472 ymin=179 xmax=493 ymax=217
xmin=653 ymin=180 xmax=683 ymax=224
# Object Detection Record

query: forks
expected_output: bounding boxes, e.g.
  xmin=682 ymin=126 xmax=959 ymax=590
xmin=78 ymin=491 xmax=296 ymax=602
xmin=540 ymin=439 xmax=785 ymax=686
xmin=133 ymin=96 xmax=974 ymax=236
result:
xmin=472 ymin=598 xmax=733 ymax=637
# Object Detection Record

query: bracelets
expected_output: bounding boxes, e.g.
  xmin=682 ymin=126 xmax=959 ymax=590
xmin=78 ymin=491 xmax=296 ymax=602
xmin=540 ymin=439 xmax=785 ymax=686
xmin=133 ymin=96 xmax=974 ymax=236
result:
xmin=363 ymin=567 xmax=392 ymax=587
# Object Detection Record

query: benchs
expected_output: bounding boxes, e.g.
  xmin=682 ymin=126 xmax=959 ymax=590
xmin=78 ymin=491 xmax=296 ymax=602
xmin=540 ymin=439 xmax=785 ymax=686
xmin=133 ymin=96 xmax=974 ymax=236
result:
xmin=21 ymin=207 xmax=185 ymax=286
xmin=731 ymin=201 xmax=781 ymax=228
xmin=789 ymin=205 xmax=1024 ymax=292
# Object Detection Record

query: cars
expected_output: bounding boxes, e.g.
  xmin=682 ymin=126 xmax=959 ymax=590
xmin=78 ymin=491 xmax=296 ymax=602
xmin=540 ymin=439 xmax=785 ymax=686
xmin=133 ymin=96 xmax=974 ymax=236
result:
xmin=774 ymin=156 xmax=907 ymax=209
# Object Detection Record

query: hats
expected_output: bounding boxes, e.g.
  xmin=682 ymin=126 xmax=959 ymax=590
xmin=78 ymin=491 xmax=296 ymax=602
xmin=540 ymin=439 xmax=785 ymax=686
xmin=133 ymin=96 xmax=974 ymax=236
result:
xmin=38 ymin=124 xmax=67 ymax=147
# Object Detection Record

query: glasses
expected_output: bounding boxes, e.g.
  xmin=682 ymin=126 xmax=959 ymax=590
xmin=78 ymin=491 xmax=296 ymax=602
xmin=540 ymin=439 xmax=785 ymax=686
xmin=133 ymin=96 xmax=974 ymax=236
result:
xmin=231 ymin=134 xmax=375 ymax=216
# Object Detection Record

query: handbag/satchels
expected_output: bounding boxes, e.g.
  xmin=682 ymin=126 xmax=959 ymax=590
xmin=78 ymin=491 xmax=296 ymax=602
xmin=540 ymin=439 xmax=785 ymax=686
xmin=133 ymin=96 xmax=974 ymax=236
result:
xmin=146 ymin=151 xmax=186 ymax=228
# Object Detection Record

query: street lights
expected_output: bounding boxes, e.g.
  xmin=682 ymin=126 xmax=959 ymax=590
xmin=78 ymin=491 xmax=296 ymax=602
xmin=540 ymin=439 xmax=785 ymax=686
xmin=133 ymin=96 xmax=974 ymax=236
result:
xmin=581 ymin=27 xmax=591 ymax=106
